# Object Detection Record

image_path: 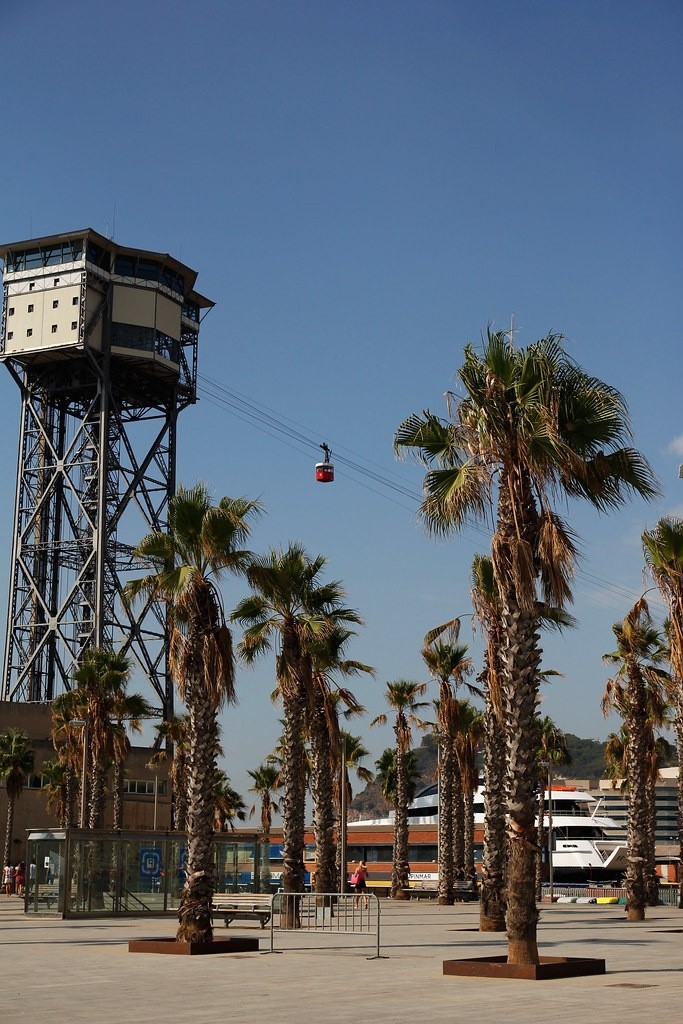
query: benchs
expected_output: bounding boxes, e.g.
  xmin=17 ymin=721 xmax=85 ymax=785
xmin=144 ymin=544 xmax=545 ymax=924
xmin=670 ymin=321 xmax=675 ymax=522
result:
xmin=211 ymin=892 xmax=274 ymax=929
xmin=402 ymin=880 xmax=477 ymax=903
xmin=21 ymin=883 xmax=77 ymax=909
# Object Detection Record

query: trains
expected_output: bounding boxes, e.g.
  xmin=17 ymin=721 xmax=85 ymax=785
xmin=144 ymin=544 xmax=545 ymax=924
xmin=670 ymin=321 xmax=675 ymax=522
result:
xmin=213 ymin=823 xmax=549 ymax=901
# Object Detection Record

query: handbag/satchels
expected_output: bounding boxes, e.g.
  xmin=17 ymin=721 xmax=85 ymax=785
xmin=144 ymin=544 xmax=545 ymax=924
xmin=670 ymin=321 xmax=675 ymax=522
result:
xmin=351 ymin=869 xmax=360 ymax=884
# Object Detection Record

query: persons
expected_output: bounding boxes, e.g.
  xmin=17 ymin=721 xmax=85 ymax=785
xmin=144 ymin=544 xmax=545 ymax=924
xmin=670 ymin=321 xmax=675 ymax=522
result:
xmin=13 ymin=860 xmax=26 ymax=895
xmin=355 ymin=860 xmax=369 ymax=909
xmin=2 ymin=860 xmax=16 ymax=897
xmin=46 ymin=863 xmax=54 ymax=884
xmin=29 ymin=858 xmax=36 ymax=891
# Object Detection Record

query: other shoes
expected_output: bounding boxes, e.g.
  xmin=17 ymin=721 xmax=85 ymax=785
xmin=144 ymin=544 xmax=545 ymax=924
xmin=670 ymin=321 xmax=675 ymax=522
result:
xmin=354 ymin=906 xmax=358 ymax=908
xmin=365 ymin=904 xmax=367 ymax=909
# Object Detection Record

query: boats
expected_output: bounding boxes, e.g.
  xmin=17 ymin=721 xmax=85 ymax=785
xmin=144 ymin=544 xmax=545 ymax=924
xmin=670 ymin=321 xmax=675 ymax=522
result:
xmin=347 ymin=775 xmax=627 ymax=888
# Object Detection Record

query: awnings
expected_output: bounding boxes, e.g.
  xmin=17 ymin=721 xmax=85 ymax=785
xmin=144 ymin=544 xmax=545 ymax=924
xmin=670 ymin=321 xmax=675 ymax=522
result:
xmin=248 ymin=843 xmax=285 ymax=860
xmin=225 ymin=870 xmax=313 ymax=887
